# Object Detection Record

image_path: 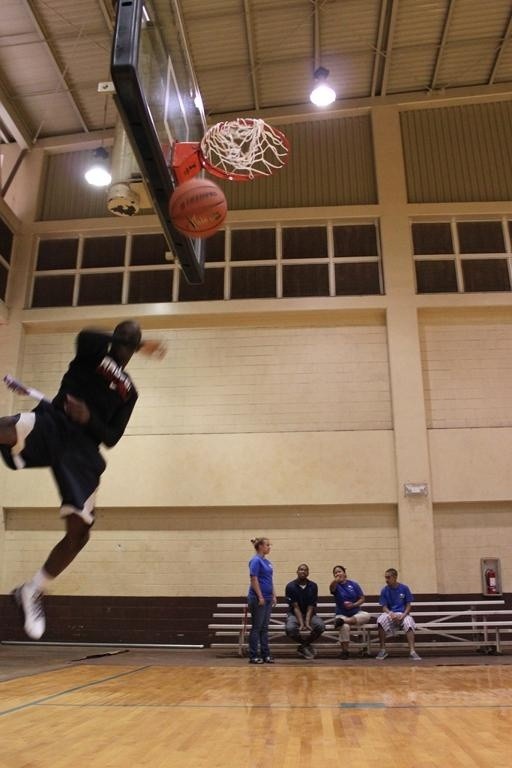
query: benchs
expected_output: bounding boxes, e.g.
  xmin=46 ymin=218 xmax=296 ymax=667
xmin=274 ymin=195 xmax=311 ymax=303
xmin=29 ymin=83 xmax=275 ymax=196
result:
xmin=207 ymin=592 xmax=512 ymax=659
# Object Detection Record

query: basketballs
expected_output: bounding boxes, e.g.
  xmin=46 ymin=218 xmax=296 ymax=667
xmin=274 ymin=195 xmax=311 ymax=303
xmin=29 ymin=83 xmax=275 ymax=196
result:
xmin=169 ymin=177 xmax=227 ymax=239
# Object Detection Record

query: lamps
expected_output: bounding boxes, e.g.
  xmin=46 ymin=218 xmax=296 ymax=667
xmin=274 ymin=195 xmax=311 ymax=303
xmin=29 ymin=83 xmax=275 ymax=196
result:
xmin=82 ymin=89 xmax=116 ymax=188
xmin=309 ymin=1 xmax=338 ymax=110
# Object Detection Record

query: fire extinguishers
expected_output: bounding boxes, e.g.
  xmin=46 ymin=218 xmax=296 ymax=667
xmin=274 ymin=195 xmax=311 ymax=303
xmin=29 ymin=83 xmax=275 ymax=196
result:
xmin=485 ymin=568 xmax=496 ymax=594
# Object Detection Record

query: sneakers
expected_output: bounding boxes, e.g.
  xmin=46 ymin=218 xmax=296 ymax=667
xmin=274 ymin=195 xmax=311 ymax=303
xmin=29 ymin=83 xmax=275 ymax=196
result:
xmin=340 ymin=652 xmax=350 ymax=658
xmin=249 ymin=658 xmax=263 ymax=663
xmin=298 ymin=644 xmax=315 ymax=659
xmin=409 ymin=653 xmax=421 ymax=661
xmin=12 ymin=583 xmax=46 ymax=641
xmin=264 ymin=656 xmax=275 ymax=663
xmin=334 ymin=618 xmax=344 ymax=629
xmin=377 ymin=651 xmax=389 ymax=660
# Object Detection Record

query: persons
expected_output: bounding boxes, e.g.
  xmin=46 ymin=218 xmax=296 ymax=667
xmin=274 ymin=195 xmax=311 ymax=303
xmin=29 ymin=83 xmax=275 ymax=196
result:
xmin=1 ymin=321 xmax=165 ymax=641
xmin=376 ymin=569 xmax=423 ymax=661
xmin=285 ymin=564 xmax=325 ymax=660
xmin=247 ymin=536 xmax=277 ymax=664
xmin=329 ymin=566 xmax=371 ymax=660
